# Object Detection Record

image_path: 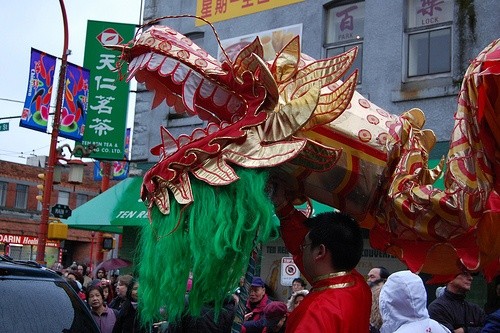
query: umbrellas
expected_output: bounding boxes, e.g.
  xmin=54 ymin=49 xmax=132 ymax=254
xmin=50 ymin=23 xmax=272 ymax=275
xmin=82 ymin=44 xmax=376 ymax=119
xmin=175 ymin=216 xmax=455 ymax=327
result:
xmin=91 ymin=258 xmax=132 ymax=280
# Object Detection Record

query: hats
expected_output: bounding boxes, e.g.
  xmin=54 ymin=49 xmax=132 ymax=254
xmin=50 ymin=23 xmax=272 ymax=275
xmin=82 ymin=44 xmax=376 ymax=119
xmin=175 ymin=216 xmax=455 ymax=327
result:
xmin=250 ymin=276 xmax=266 ymax=287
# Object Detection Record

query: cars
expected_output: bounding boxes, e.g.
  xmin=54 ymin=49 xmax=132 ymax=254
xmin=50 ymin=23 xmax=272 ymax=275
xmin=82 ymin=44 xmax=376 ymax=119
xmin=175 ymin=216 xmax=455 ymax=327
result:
xmin=0 ymin=254 xmax=103 ymax=333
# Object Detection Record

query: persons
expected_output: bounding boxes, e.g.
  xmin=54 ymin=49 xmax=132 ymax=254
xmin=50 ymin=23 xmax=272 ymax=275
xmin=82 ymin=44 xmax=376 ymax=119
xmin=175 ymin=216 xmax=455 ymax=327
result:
xmin=85 ymin=285 xmax=120 ymax=333
xmin=64 ymin=263 xmax=500 ymax=333
xmin=379 ymin=270 xmax=452 ymax=333
xmin=264 ymin=180 xmax=372 ymax=333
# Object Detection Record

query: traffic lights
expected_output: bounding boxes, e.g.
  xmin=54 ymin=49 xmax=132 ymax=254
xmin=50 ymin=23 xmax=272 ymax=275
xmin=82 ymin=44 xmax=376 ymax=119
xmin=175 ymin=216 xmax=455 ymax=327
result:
xmin=35 ymin=173 xmax=44 ymax=201
